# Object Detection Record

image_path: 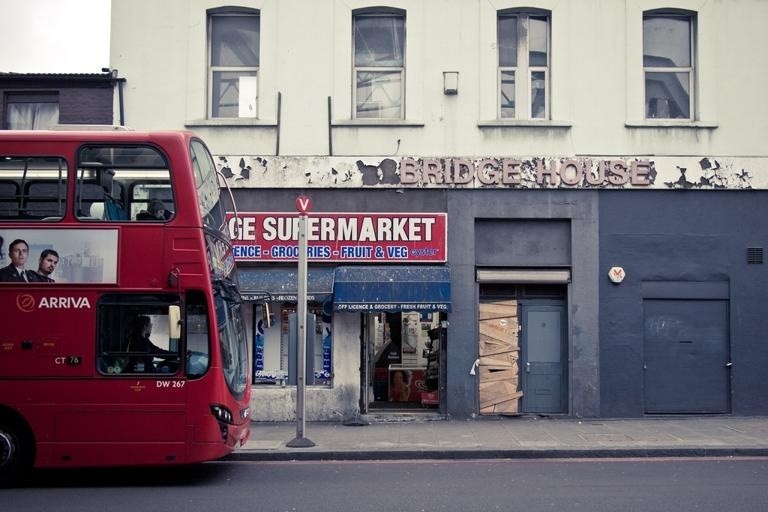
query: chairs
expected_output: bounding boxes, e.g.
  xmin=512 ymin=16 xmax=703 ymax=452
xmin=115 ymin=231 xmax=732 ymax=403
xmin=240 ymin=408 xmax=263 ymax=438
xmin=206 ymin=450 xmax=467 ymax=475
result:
xmin=104 ymin=193 xmax=120 ymax=220
xmin=115 ymin=199 xmax=128 ymax=220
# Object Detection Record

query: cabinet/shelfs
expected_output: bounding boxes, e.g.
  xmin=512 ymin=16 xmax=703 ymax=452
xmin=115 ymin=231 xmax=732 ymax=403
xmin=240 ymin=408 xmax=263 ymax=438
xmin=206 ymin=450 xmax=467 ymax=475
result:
xmin=421 ymin=327 xmax=439 ymax=408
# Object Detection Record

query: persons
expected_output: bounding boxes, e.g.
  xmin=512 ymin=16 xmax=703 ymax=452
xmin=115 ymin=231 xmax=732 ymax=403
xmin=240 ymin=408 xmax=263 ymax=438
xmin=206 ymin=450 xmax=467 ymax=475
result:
xmin=390 ymin=370 xmax=412 ymax=401
xmin=136 ymin=197 xmax=165 ymax=220
xmin=129 ymin=316 xmax=178 ymax=372
xmin=0 ymin=239 xmax=41 ymax=282
xmin=29 ymin=249 xmax=59 ymax=282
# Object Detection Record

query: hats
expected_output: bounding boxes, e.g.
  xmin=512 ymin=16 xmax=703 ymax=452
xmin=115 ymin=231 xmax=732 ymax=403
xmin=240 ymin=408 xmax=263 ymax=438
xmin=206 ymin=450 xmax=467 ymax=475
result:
xmin=149 ymin=200 xmax=165 ymax=210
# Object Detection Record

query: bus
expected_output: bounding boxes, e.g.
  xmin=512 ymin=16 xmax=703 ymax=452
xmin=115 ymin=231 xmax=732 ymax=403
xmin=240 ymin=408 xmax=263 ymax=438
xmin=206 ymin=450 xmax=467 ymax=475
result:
xmin=1 ymin=123 xmax=276 ymax=488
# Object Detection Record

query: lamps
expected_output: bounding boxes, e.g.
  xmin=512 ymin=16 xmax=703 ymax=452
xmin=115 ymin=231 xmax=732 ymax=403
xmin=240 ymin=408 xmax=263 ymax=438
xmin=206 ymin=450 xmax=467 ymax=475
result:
xmin=443 ymin=72 xmax=459 ymax=94
